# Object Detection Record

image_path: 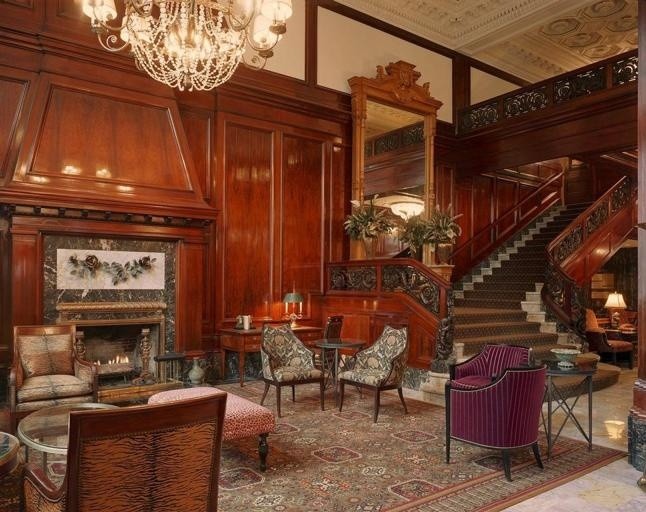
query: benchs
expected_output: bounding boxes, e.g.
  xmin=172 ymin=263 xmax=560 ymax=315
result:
xmin=148 ymin=386 xmax=277 ymax=472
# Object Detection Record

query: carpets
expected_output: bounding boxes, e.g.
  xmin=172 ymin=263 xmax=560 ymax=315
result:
xmin=0 ymin=373 xmax=632 ymax=512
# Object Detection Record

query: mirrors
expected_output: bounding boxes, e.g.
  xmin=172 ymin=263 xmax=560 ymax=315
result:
xmin=349 ymin=58 xmax=437 ymax=265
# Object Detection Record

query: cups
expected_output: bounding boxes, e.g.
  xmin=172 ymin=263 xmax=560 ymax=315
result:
xmin=242 ymin=315 xmax=252 ymax=330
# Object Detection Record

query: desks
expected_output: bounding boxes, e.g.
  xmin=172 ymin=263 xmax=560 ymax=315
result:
xmin=535 ymin=356 xmax=594 ymax=457
xmin=309 ymin=338 xmax=367 ymax=413
xmin=218 ymin=326 xmax=324 ymax=389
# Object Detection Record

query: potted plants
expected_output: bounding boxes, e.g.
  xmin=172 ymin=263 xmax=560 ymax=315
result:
xmin=425 ymin=204 xmax=466 ymax=265
xmin=396 ymin=211 xmax=424 ymax=261
xmin=345 ymin=199 xmax=391 ymax=258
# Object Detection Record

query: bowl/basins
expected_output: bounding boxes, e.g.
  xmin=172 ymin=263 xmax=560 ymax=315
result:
xmin=551 ymin=348 xmax=583 ymax=361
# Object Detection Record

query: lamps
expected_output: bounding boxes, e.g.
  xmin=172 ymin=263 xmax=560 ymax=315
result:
xmin=78 ymin=0 xmax=294 ymax=95
xmin=283 ymin=292 xmax=304 ymax=328
xmin=603 ymin=291 xmax=628 ymax=326
xmin=387 ymin=199 xmax=424 ymax=223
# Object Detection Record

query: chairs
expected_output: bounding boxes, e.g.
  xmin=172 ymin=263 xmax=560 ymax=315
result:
xmin=449 ymin=344 xmax=533 ymax=389
xmin=444 ymin=361 xmax=550 ymax=481
xmin=309 ymin=315 xmax=345 ymax=370
xmin=19 ymin=390 xmax=229 ymax=511
xmin=584 ymin=306 xmax=637 ymax=372
xmin=257 ymin=323 xmax=332 ymax=417
xmin=5 ymin=322 xmax=99 ymax=432
xmin=337 ymin=322 xmax=412 ymax=423
